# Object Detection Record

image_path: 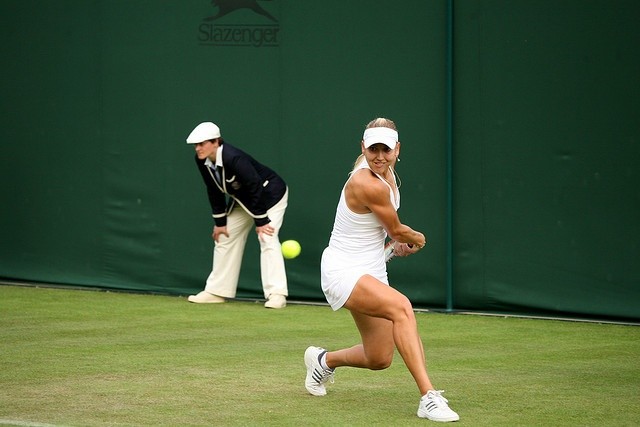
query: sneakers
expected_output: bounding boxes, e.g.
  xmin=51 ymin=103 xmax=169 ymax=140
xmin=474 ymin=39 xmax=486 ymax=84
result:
xmin=264 ymin=295 xmax=288 ymax=309
xmin=418 ymin=391 xmax=460 ymax=424
xmin=304 ymin=346 xmax=334 ymax=397
xmin=188 ymin=291 xmax=226 ymax=304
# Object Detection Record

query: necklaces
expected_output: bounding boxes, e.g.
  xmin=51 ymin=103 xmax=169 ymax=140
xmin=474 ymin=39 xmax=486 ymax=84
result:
xmin=383 ymin=167 xmax=402 ymax=189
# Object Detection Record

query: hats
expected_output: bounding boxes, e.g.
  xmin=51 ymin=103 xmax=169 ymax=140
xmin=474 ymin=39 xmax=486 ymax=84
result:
xmin=363 ymin=127 xmax=400 ymax=150
xmin=187 ymin=121 xmax=221 ymax=145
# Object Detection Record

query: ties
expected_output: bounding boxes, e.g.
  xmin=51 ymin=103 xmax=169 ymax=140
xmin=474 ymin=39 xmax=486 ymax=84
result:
xmin=213 ymin=167 xmax=223 ymax=186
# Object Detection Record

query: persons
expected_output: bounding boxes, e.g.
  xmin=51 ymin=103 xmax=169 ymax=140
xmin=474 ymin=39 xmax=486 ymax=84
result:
xmin=303 ymin=117 xmax=460 ymax=422
xmin=186 ymin=122 xmax=289 ymax=308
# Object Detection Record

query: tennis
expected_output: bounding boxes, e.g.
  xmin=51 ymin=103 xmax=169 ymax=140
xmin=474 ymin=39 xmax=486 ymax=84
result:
xmin=281 ymin=240 xmax=301 ymax=259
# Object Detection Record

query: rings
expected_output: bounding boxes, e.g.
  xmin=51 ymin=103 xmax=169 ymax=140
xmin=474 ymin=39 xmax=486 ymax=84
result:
xmin=266 ymin=228 xmax=269 ymax=231
